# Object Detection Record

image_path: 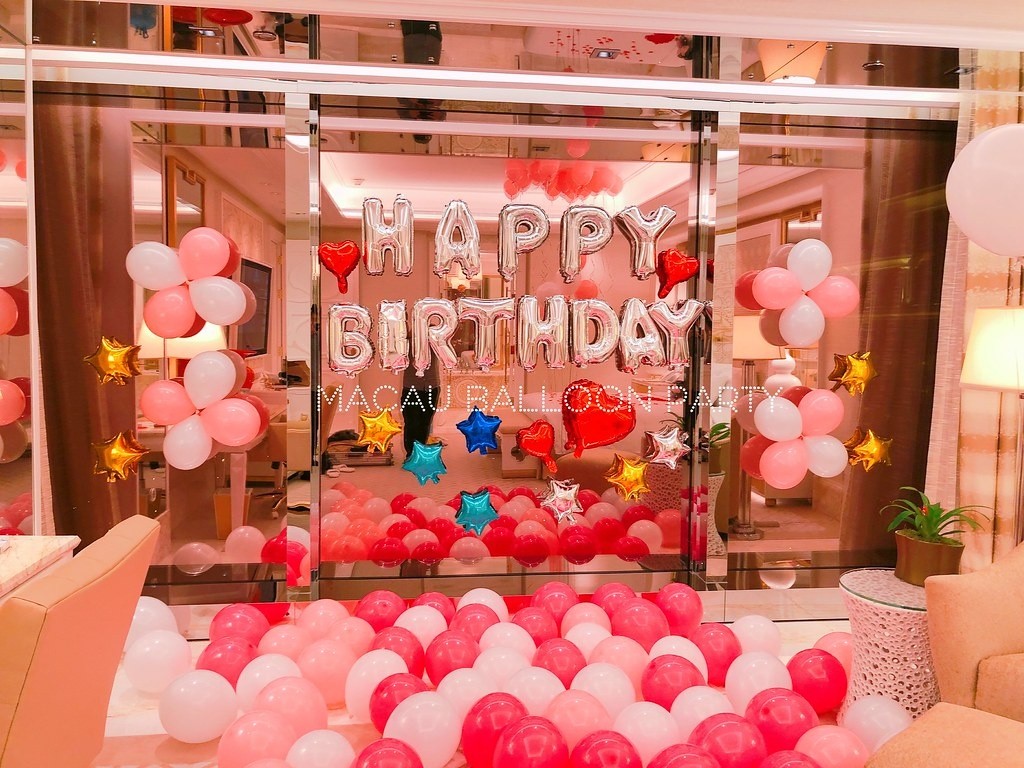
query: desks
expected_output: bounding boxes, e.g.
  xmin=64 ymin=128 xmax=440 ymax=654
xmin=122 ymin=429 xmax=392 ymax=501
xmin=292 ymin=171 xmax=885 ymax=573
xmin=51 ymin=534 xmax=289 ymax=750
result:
xmin=633 ymin=455 xmax=728 ymax=557
xmin=131 ymin=403 xmax=289 ymax=542
xmin=835 ymin=568 xmax=941 ymax=726
xmin=0 ymin=534 xmax=81 ymax=607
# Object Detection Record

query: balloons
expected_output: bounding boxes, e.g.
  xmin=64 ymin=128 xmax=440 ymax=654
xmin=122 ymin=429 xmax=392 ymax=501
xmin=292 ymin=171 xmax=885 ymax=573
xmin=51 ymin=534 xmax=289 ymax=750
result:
xmin=735 ymin=386 xmax=811 ymax=481
xmin=122 ymin=429 xmax=152 ymax=477
xmin=89 ymin=432 xmax=143 ymax=482
xmin=165 ymin=235 xmax=258 ymax=340
xmin=121 ymin=581 xmax=912 ymax=768
xmin=82 ymin=335 xmax=132 ymax=385
xmin=110 ymin=337 xmax=141 ymax=383
xmin=750 ymin=238 xmax=861 ymax=348
xmin=734 ymin=243 xmax=795 ymax=347
xmin=125 ymin=226 xmax=247 ymax=339
xmin=223 ymin=483 xmax=682 ymax=568
xmin=141 ymin=351 xmax=261 ymax=470
xmin=828 ymin=352 xmax=852 ymax=393
xmin=754 ymin=351 xmax=892 ymax=489
xmin=170 ymin=349 xmax=272 ymax=462
xmin=844 ymin=426 xmax=867 ymax=468
xmin=945 ymin=124 xmax=1023 ymax=257
xmin=0 ymin=148 xmax=34 ymax=536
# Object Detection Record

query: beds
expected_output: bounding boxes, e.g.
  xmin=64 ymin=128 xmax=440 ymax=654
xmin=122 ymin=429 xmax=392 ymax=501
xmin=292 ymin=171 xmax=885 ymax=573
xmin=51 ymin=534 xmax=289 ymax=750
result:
xmin=486 ymin=389 xmax=685 ymax=479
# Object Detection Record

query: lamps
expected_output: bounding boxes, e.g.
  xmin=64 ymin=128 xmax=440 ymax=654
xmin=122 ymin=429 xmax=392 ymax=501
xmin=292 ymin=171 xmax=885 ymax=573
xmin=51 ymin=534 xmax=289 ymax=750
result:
xmin=959 ymin=306 xmax=1024 ymax=406
xmin=732 ymin=316 xmax=786 ymax=539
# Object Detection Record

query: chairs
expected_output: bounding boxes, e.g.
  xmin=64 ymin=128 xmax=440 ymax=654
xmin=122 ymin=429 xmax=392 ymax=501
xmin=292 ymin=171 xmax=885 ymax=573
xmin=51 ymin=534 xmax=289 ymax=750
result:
xmin=2 ymin=514 xmax=161 ymax=767
xmin=250 ymin=385 xmax=343 ymax=521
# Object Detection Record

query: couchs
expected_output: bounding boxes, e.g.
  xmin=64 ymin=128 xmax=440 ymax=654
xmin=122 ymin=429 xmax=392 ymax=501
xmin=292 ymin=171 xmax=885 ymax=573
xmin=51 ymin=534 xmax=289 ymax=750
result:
xmin=864 ymin=538 xmax=1023 ymax=768
xmin=708 ymin=433 xmax=743 ymax=543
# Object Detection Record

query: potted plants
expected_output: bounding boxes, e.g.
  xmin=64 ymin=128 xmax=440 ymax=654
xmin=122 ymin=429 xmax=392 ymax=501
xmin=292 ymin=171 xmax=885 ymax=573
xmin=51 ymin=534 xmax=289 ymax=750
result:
xmin=656 ymin=410 xmax=732 ymax=465
xmin=878 ymin=485 xmax=998 ymax=589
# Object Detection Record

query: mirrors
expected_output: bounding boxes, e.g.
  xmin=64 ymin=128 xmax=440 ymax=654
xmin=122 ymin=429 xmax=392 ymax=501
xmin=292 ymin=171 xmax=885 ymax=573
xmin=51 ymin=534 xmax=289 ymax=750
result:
xmin=3 ymin=0 xmax=970 ymax=593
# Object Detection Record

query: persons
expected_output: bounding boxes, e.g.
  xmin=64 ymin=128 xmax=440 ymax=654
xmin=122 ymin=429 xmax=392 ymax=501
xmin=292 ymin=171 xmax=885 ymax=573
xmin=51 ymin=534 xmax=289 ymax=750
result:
xmin=395 ymin=19 xmax=446 ymax=148
xmin=320 ymin=198 xmax=705 ymax=537
xmin=402 ymin=325 xmax=441 ymax=464
